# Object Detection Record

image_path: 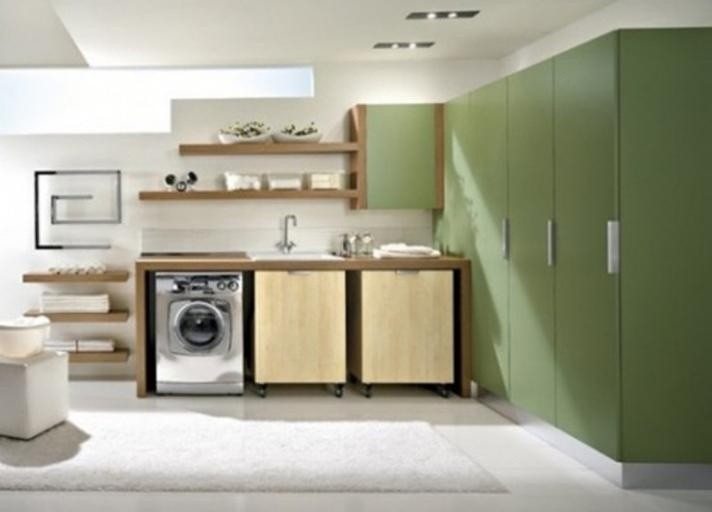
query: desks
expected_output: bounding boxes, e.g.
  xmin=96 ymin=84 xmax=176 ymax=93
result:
xmin=135 ymin=255 xmax=473 ymax=397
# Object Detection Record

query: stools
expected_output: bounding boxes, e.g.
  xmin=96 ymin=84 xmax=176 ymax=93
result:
xmin=1 ymin=351 xmax=69 ymax=441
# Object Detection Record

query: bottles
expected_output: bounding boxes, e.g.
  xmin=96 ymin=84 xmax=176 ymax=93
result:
xmin=339 ymin=232 xmax=352 ymax=258
xmin=361 ymin=232 xmax=374 ymax=256
xmin=349 ymin=233 xmax=361 ymax=258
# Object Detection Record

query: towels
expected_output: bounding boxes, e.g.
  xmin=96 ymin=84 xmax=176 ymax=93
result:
xmin=38 ymin=290 xmax=110 ymax=314
xmin=42 ymin=338 xmax=115 ymax=354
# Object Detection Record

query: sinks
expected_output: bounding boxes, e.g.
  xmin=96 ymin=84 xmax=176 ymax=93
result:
xmin=249 ymin=252 xmax=347 ymax=262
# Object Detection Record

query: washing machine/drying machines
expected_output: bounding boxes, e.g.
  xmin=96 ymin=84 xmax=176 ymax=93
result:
xmin=154 ymin=271 xmax=245 ymax=395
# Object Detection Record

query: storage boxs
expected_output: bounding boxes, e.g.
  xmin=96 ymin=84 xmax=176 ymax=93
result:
xmin=0 ymin=318 xmax=51 ymax=359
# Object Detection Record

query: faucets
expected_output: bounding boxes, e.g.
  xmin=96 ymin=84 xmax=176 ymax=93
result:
xmin=277 ymin=215 xmax=298 ymax=253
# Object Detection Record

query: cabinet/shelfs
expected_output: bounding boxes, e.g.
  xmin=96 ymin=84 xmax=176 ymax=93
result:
xmin=20 ymin=270 xmax=130 ymax=363
xmin=437 ymin=25 xmax=711 ymax=487
xmin=136 ymin=142 xmax=357 ymax=202
xmin=349 ymin=103 xmax=443 ymax=211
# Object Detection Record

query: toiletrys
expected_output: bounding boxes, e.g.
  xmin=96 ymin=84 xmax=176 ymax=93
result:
xmin=339 ymin=232 xmax=373 ymax=258
